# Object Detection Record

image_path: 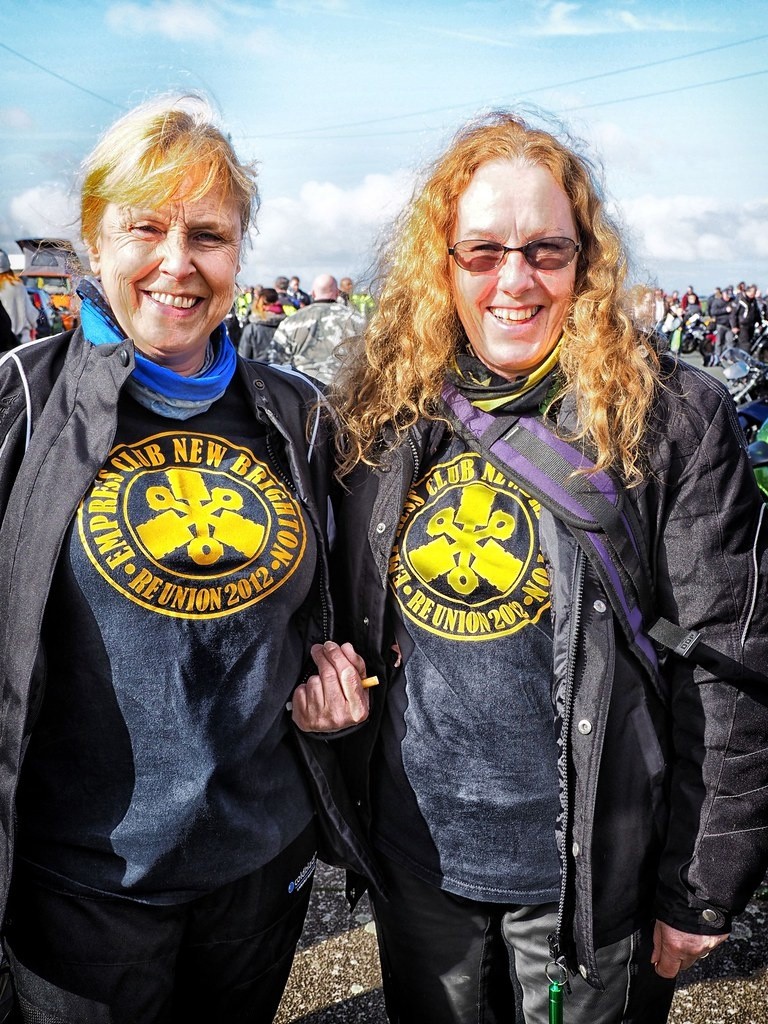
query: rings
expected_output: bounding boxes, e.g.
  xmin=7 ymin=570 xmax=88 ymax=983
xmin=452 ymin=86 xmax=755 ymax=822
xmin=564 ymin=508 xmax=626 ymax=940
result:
xmin=701 ymin=952 xmax=709 ymax=959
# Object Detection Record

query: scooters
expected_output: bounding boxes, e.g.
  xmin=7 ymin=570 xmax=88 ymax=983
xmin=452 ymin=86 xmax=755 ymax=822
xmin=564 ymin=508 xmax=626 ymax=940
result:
xmin=680 ymin=312 xmax=768 ymax=499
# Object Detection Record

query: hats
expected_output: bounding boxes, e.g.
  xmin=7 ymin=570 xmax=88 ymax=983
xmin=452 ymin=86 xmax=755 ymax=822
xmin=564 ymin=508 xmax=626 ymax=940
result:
xmin=0 ymin=251 xmax=10 ymax=273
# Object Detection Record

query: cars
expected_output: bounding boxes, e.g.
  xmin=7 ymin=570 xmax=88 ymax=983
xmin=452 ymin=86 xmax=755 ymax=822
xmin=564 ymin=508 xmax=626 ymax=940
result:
xmin=20 ymin=271 xmax=78 ymax=331
xmin=26 ymin=287 xmax=66 ymax=337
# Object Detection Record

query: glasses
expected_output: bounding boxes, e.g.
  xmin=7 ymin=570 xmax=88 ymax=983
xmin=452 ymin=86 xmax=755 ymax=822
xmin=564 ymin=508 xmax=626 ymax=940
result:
xmin=448 ymin=237 xmax=582 ymax=272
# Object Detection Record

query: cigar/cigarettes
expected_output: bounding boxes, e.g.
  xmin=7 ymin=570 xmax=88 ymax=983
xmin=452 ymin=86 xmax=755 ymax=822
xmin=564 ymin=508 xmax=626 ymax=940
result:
xmin=287 ymin=675 xmax=379 ymax=712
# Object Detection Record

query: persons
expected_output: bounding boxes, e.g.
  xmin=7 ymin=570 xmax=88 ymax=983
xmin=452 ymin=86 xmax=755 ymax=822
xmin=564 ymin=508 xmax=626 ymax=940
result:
xmin=0 ymin=95 xmax=768 ymax=1024
